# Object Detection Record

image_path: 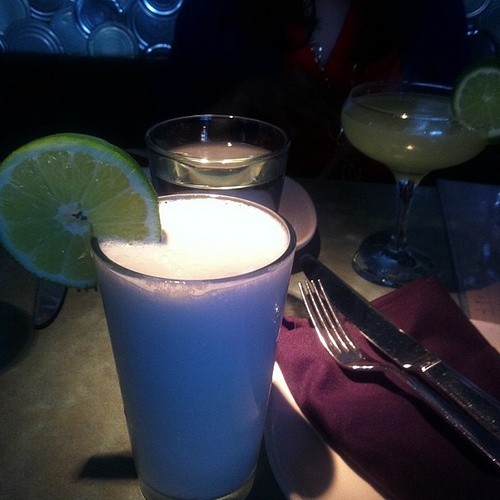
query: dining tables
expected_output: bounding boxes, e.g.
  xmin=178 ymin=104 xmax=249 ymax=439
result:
xmin=0 ymin=178 xmax=499 ymax=500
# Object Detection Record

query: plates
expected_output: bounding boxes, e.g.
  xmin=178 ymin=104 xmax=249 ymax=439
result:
xmin=136 ymin=165 xmax=317 ymax=254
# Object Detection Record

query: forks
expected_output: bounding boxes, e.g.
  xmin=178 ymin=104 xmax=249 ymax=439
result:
xmin=298 ymin=279 xmax=500 ymax=473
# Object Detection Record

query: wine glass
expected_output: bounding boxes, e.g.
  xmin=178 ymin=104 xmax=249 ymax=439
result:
xmin=340 ymin=82 xmax=488 ymax=289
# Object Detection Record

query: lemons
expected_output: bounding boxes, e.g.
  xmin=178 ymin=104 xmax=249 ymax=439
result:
xmin=0 ymin=133 xmax=162 ymax=288
xmin=450 ymin=66 xmax=500 ymax=137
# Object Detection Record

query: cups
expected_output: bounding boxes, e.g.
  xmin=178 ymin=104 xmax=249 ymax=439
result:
xmin=144 ymin=115 xmax=289 ymax=214
xmin=90 ymin=193 xmax=297 ymax=500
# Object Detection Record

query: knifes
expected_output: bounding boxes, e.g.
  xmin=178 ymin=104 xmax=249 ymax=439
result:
xmin=298 ymin=254 xmax=500 ymax=442
